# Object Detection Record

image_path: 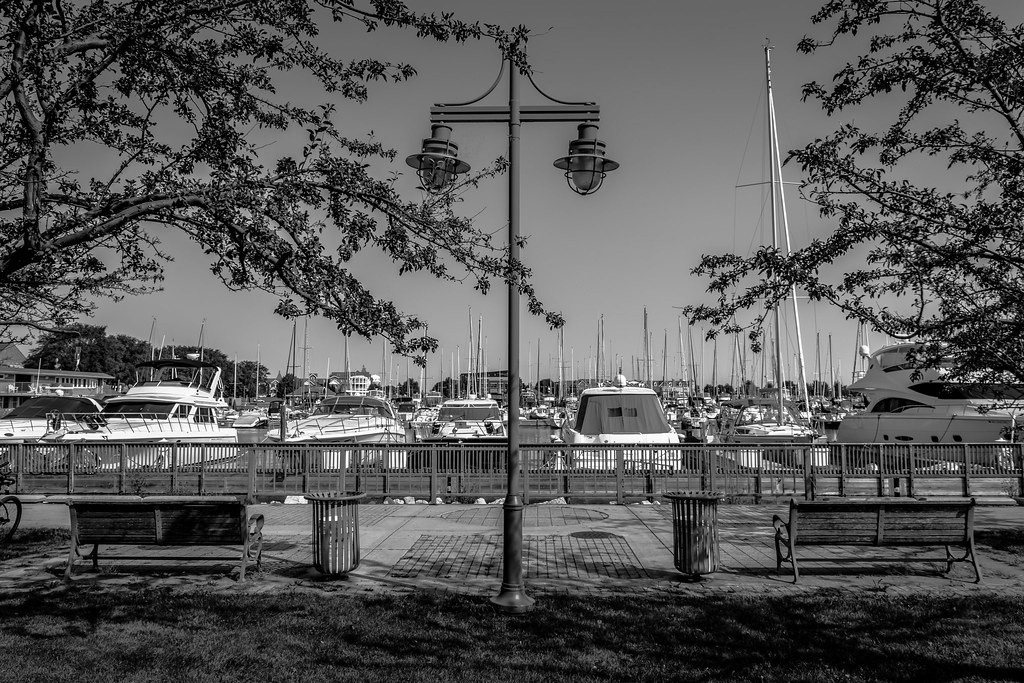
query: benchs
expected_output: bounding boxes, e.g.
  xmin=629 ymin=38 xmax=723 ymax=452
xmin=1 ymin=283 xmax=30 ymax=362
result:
xmin=771 ymin=498 xmax=980 ymax=585
xmin=67 ymin=497 xmax=264 ymax=584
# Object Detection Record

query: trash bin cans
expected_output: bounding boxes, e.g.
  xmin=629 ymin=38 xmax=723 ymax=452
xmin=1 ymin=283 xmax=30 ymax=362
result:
xmin=661 ymin=491 xmax=727 ymax=575
xmin=304 ymin=490 xmax=367 ymax=575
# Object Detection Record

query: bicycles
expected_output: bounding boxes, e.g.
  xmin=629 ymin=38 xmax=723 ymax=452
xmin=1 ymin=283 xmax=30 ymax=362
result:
xmin=0 ymin=461 xmax=22 ymax=545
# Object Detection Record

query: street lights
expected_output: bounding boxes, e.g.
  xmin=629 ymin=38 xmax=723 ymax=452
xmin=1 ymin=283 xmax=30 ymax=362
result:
xmin=405 ymin=34 xmax=620 ymax=619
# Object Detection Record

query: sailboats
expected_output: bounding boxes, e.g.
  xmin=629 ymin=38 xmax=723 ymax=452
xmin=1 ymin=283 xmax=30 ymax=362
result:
xmin=0 ymin=38 xmax=1024 ymax=472
